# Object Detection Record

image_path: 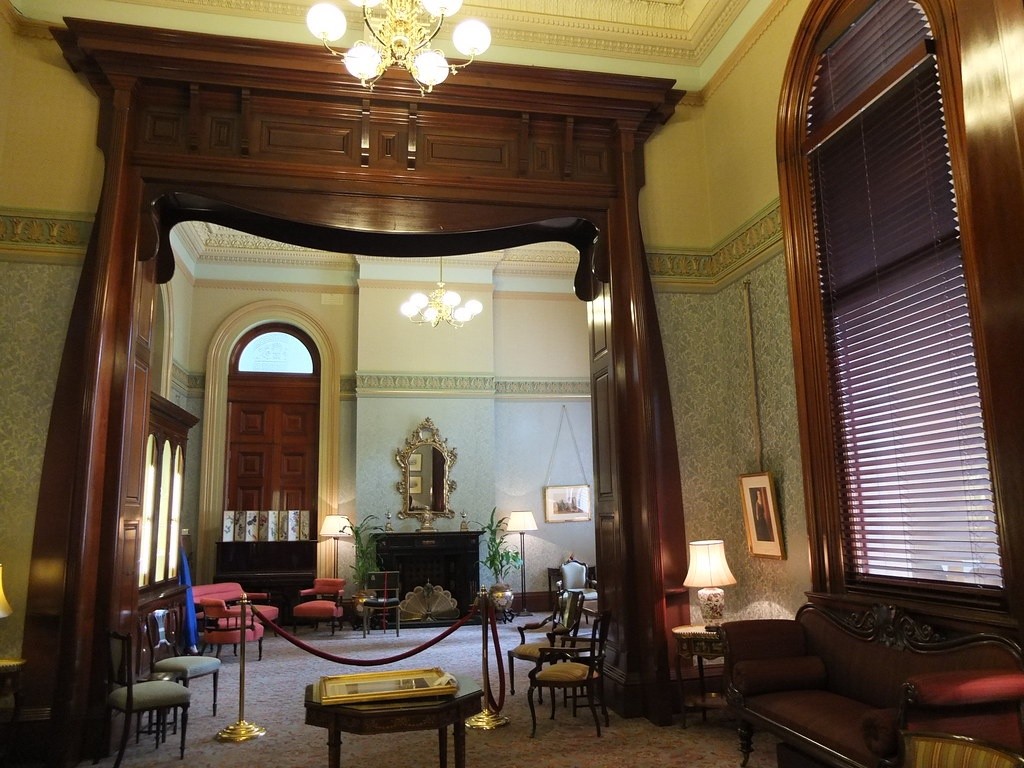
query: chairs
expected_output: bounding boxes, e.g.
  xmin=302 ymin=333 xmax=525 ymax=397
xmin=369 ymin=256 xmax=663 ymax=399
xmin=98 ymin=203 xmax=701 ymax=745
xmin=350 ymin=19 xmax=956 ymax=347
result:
xmin=507 ymin=556 xmax=610 ymax=737
xmin=93 ymin=578 xmax=346 ymax=768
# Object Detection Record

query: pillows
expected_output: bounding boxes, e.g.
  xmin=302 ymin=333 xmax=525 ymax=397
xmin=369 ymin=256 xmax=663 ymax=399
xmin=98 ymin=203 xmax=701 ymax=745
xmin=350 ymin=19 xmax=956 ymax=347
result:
xmin=735 ymin=656 xmax=825 ymax=694
xmin=861 ymin=707 xmax=897 ymax=758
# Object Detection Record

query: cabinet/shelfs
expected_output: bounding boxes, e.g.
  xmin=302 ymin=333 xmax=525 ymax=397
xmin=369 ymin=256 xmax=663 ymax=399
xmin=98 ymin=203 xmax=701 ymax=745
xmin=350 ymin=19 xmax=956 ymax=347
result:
xmin=369 ymin=530 xmax=486 ymax=623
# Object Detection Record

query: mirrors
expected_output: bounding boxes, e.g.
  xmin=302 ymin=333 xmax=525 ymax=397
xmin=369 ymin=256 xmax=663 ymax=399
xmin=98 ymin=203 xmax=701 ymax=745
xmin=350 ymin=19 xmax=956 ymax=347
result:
xmin=395 ymin=417 xmax=458 ymax=521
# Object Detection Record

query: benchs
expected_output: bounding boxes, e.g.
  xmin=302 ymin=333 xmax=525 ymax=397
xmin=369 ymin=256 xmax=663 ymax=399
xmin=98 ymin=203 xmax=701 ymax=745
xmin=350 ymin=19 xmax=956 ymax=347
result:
xmin=725 ymin=600 xmax=1024 ymax=768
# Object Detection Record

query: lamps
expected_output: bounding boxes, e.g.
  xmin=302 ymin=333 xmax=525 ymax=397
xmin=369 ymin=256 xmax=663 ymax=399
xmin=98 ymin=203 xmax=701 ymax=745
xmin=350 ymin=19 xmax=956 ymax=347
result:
xmin=683 ymin=538 xmax=735 ymax=631
xmin=320 ymin=515 xmax=354 ymax=626
xmin=401 ymin=257 xmax=482 ymax=327
xmin=305 ymin=0 xmax=491 ymax=93
xmin=507 ymin=511 xmax=539 ymax=616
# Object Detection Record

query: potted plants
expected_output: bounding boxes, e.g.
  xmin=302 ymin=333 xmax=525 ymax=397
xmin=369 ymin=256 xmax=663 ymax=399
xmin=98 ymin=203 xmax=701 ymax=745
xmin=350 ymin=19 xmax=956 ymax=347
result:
xmin=471 ymin=506 xmax=523 ymax=613
xmin=342 ymin=515 xmax=384 ymax=617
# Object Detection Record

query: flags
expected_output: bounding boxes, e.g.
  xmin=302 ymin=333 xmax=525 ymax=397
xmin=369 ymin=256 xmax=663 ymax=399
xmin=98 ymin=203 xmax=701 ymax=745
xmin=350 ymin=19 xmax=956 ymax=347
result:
xmin=179 ymin=547 xmax=198 ymax=654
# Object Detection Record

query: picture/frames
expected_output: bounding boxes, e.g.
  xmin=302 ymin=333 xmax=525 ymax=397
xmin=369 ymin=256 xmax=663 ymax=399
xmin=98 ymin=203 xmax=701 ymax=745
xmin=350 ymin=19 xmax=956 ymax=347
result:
xmin=738 ymin=471 xmax=787 ymax=560
xmin=544 ymin=485 xmax=592 ymax=522
xmin=409 ymin=476 xmax=422 ymax=494
xmin=407 ymin=453 xmax=422 ymax=472
xmin=317 ymin=666 xmax=459 ymax=707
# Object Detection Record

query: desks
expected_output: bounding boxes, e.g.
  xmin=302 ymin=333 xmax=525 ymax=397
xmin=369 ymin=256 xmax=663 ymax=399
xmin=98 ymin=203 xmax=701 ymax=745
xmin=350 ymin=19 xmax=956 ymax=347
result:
xmin=304 ymin=676 xmax=484 ymax=768
xmin=674 ymin=626 xmax=730 ymax=729
